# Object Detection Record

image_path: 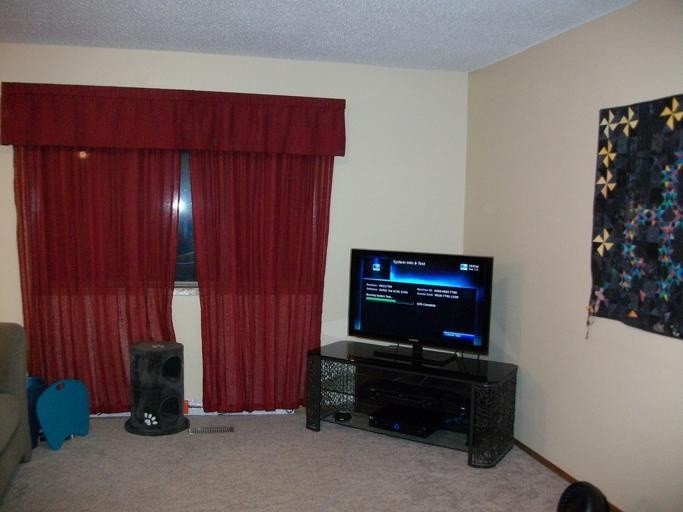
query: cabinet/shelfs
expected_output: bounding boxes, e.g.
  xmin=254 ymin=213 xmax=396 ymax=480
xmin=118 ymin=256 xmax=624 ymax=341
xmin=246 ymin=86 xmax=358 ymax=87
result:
xmin=306 ymin=341 xmax=519 ymax=468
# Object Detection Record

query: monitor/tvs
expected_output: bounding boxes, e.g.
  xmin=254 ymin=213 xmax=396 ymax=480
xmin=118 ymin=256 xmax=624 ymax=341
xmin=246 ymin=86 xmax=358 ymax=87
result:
xmin=348 ymin=246 xmax=493 ymax=366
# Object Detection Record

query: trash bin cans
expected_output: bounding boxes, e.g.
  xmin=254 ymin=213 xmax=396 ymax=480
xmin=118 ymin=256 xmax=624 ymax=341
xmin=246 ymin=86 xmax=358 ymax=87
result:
xmin=124 ymin=341 xmax=190 ymax=437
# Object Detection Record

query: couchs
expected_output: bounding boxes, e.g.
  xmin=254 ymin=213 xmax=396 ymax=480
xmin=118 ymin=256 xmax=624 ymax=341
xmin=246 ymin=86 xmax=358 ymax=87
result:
xmin=0 ymin=322 xmax=32 ymax=495
